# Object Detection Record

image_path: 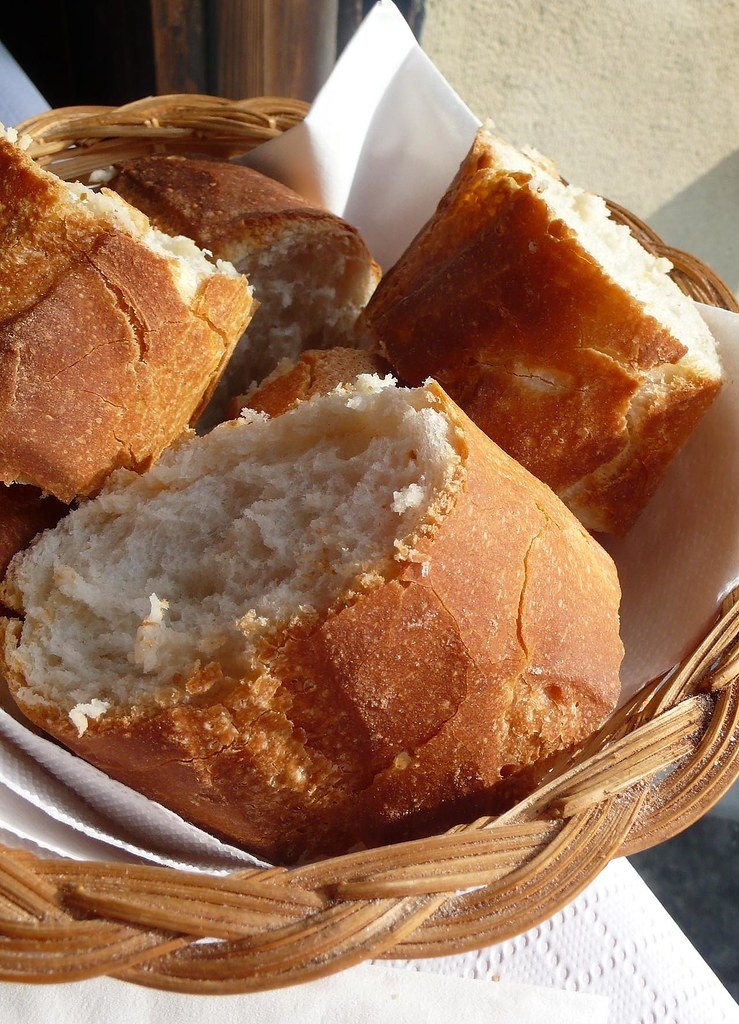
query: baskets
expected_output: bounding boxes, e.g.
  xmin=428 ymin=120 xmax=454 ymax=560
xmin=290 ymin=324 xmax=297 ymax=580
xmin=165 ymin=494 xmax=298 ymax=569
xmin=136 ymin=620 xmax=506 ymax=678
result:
xmin=0 ymin=94 xmax=739 ymax=997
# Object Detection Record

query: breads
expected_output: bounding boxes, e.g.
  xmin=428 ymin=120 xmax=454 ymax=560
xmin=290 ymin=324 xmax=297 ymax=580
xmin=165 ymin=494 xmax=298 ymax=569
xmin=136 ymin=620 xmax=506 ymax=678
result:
xmin=199 ymin=346 xmax=404 ymax=438
xmin=1 ymin=371 xmax=622 ymax=866
xmin=81 ymin=156 xmax=383 ymax=433
xmin=0 ymin=483 xmax=74 ymax=586
xmin=0 ymin=119 xmax=263 ymax=506
xmin=346 ymin=119 xmax=725 ymax=552
